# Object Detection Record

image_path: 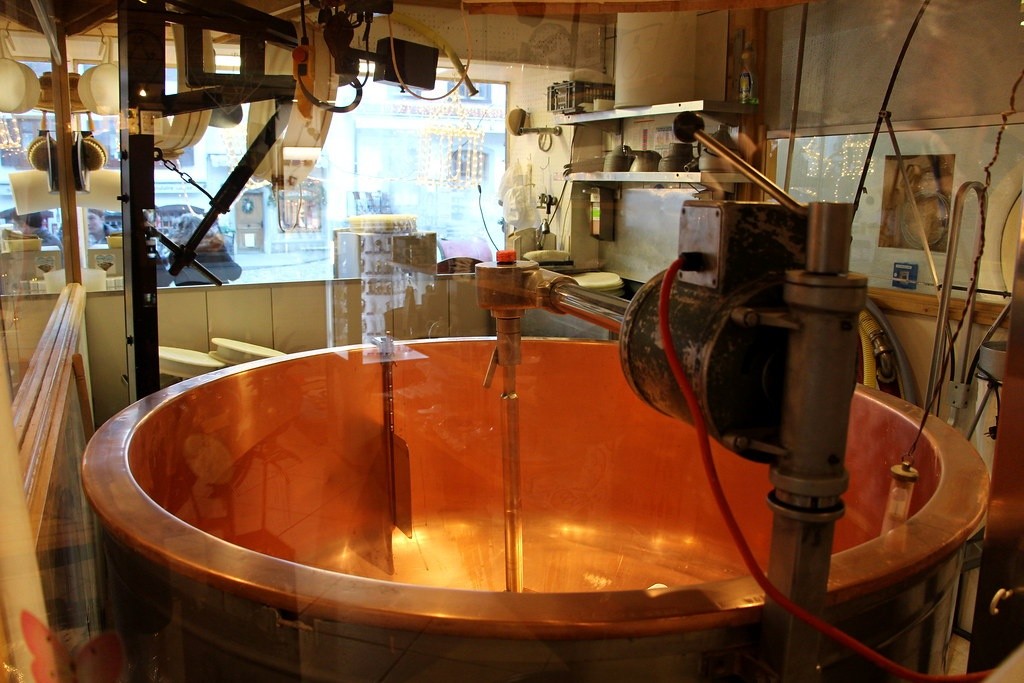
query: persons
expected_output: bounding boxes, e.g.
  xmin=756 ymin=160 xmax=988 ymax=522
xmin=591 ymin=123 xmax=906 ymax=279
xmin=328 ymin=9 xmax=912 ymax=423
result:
xmin=12 ymin=206 xmax=64 ymax=271
xmin=86 ymin=207 xmax=121 ymax=253
xmin=168 ymin=212 xmax=243 ymax=287
xmin=143 ymin=205 xmax=162 ymax=233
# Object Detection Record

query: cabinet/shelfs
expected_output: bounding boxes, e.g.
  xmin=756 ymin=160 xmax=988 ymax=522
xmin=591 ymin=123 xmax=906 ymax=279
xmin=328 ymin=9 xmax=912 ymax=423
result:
xmin=555 ymin=99 xmax=756 ymax=182
